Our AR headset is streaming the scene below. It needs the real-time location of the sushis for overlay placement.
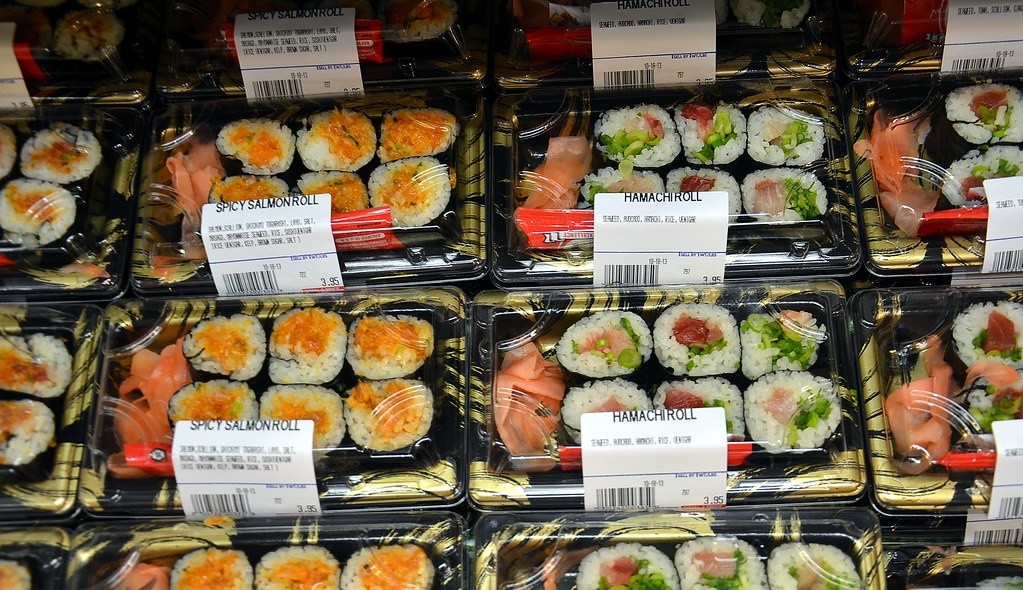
[0,0,1023,590]
